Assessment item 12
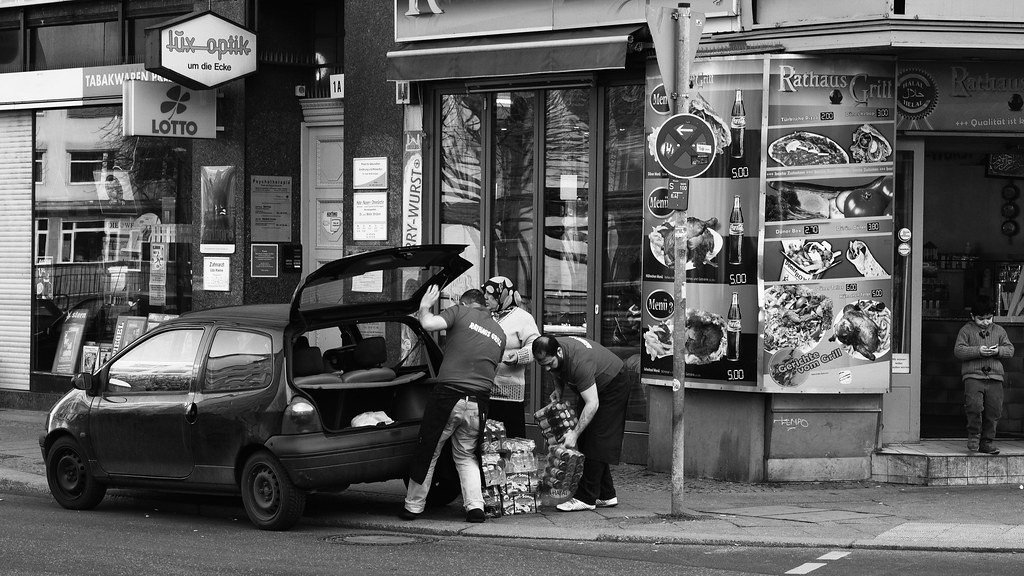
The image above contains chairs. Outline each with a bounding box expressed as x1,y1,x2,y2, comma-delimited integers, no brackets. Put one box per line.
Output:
290,335,387,376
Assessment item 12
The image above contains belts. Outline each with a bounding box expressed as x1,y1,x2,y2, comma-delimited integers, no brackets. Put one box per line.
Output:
461,395,479,403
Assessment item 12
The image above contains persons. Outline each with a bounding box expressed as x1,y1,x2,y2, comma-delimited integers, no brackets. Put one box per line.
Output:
532,334,631,511
953,294,1016,454
447,275,542,506
398,284,507,523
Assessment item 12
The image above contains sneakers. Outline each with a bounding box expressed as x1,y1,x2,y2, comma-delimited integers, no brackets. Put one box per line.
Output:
596,497,619,507
967,433,980,451
980,442,1000,454
556,498,596,511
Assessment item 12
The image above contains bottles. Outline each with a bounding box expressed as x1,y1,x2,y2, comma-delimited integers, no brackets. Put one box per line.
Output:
729,195,744,264
480,419,537,519
534,401,579,446
731,89,746,158
726,292,742,361
544,446,584,493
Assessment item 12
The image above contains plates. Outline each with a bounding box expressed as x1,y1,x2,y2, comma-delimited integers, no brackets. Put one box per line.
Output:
834,300,890,360
650,224,723,270
763,285,826,355
768,132,849,169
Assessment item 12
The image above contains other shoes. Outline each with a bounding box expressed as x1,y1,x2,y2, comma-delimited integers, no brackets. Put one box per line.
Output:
396,504,417,520
465,509,486,523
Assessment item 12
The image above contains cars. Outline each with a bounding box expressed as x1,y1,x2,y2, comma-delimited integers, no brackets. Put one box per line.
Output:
39,242,473,530
32,289,192,373
